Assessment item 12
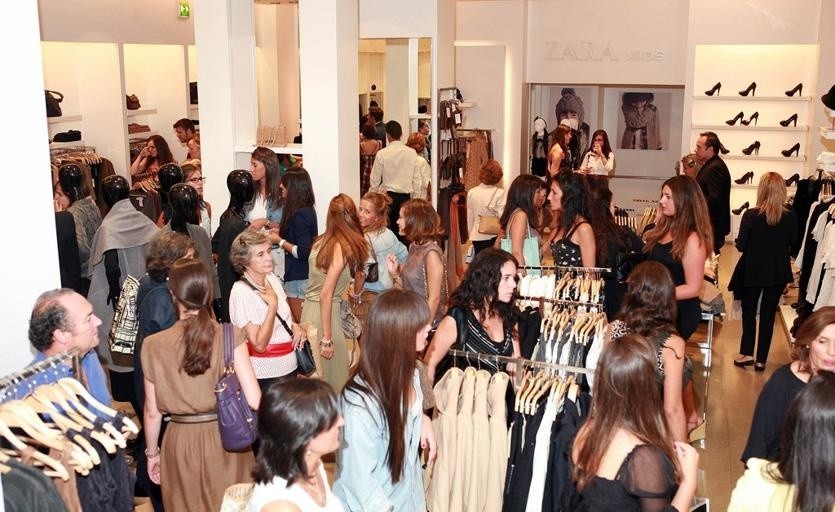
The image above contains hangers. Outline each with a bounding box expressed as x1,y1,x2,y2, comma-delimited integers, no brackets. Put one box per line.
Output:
614,206,658,233
50,144,102,167
442,267,608,424
130,170,158,195
0,350,140,483
817,171,835,225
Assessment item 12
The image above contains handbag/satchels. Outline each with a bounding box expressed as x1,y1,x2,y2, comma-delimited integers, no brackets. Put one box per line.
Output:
477,214,502,236
123,90,143,109
219,481,256,512
615,251,651,284
686,493,711,511
335,305,362,340
349,237,378,284
213,319,266,451
290,334,317,375
500,211,544,279
46,84,72,120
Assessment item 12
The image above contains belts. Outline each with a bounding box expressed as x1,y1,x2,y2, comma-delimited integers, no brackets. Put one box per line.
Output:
162,407,216,431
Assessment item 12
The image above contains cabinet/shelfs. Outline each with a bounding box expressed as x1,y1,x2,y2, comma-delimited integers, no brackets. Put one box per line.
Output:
127,109,160,140
692,96,812,218
47,113,86,149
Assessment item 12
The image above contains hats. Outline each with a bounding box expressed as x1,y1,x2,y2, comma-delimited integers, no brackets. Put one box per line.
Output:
556,88,585,131
821,84,834,111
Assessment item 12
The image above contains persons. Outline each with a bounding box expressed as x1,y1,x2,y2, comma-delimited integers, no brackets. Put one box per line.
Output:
3,101,835,512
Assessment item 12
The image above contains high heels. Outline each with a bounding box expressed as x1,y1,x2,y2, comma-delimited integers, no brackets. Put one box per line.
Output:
726,81,762,217
779,79,803,213
705,80,724,100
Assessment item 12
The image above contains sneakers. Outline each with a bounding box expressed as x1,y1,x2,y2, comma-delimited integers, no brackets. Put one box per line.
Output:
734,353,754,367
755,360,770,371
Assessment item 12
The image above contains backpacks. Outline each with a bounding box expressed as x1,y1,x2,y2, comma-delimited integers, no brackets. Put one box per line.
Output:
103,267,172,374
